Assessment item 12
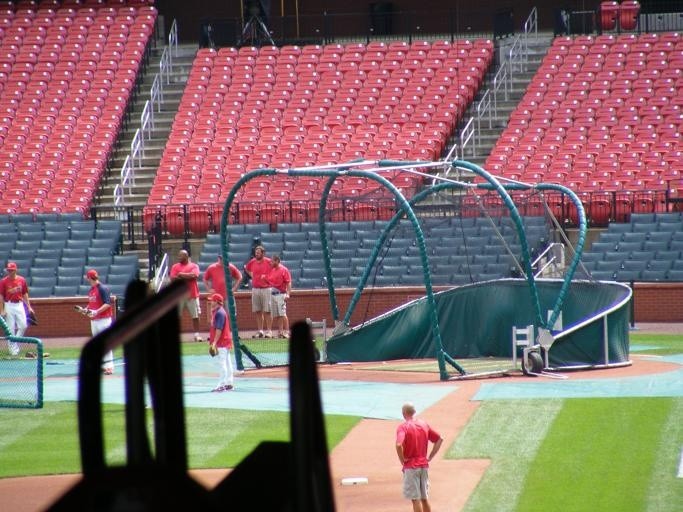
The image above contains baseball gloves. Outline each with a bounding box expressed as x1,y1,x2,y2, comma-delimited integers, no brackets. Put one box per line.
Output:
25,311,38,326
208,342,218,357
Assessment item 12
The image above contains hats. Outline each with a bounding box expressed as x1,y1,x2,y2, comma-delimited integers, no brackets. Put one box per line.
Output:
206,293,223,303
82,269,97,280
5,263,17,272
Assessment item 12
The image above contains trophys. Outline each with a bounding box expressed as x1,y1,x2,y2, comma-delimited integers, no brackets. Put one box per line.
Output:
71,304,89,317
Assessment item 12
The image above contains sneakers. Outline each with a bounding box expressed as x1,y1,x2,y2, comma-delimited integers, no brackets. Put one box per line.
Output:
193,330,291,343
211,385,234,393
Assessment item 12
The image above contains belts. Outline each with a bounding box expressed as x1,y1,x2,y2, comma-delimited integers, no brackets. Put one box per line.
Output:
271,291,285,295
3,299,20,303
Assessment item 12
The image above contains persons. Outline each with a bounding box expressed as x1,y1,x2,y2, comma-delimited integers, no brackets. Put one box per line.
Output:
262,254,292,339
168,249,204,342
79,269,114,375
0,261,36,357
241,245,276,339
205,292,235,393
394,402,444,512
201,251,242,341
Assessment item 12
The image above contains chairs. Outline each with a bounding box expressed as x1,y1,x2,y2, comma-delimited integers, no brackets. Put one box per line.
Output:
1,218,141,298
199,215,555,289
1,4,158,216
459,28,682,227
568,212,682,287
141,39,501,238
